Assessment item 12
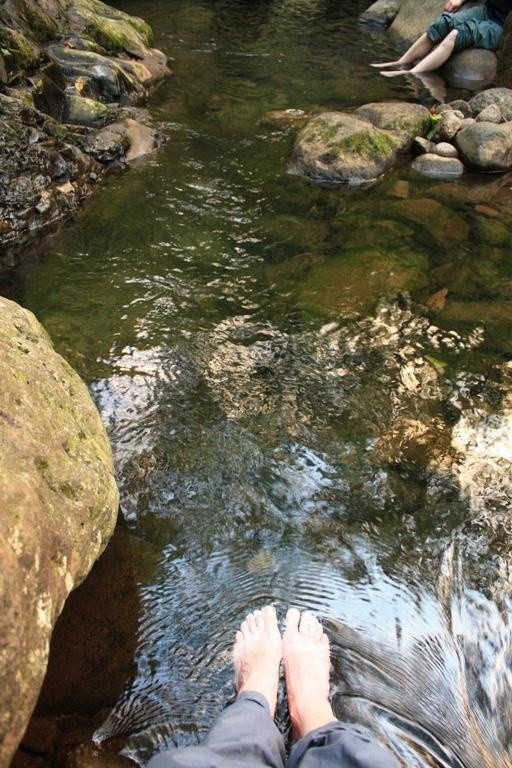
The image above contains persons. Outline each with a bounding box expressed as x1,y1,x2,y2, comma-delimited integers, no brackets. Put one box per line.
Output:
147,605,402,768
369,0,512,78
